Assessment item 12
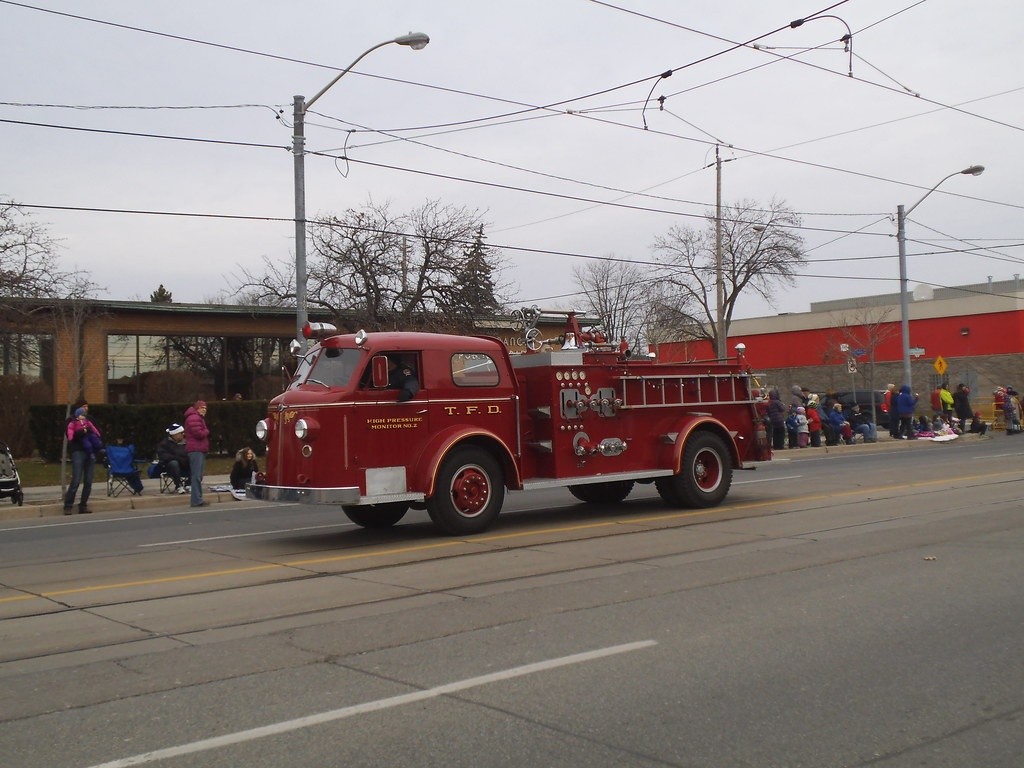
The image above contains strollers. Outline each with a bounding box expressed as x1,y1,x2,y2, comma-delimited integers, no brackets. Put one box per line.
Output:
0,442,24,507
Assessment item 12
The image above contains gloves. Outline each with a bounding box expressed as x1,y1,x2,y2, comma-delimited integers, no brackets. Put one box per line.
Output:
398,390,412,401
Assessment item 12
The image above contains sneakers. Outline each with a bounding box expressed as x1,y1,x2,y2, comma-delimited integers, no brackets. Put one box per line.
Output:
176,486,191,494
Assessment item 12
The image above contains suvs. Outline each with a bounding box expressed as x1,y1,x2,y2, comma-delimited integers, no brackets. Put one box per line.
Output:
819,389,893,429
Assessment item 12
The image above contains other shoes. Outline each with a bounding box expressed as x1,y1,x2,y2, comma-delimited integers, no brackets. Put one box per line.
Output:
864,438,876,443
907,437,918,440
90,454,95,459
191,501,209,507
980,435,988,439
64,506,72,514
1007,429,1024,434
846,439,855,445
100,449,105,453
79,506,92,513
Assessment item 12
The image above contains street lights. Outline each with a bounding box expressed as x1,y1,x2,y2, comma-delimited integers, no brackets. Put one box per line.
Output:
896,165,985,398
714,224,765,361
289,30,429,366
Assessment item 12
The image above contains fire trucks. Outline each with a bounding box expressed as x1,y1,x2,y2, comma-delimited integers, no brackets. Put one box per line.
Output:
244,305,776,536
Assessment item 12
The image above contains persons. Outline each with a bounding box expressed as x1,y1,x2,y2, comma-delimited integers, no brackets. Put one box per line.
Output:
159,422,192,494
880,383,988,439
184,400,211,507
62,400,106,515
767,385,877,450
992,384,1024,435
233,394,242,401
372,354,419,403
230,446,258,490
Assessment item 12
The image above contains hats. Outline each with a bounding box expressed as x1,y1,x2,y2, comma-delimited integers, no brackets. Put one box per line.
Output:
74,408,86,418
75,399,88,409
797,407,805,413
975,411,981,417
752,390,759,397
194,401,206,409
833,404,842,411
790,407,797,414
802,387,810,392
165,423,184,435
850,401,859,410
808,394,818,401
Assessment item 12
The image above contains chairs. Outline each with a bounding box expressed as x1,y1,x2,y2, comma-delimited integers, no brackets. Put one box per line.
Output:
160,453,200,494
103,457,143,496
